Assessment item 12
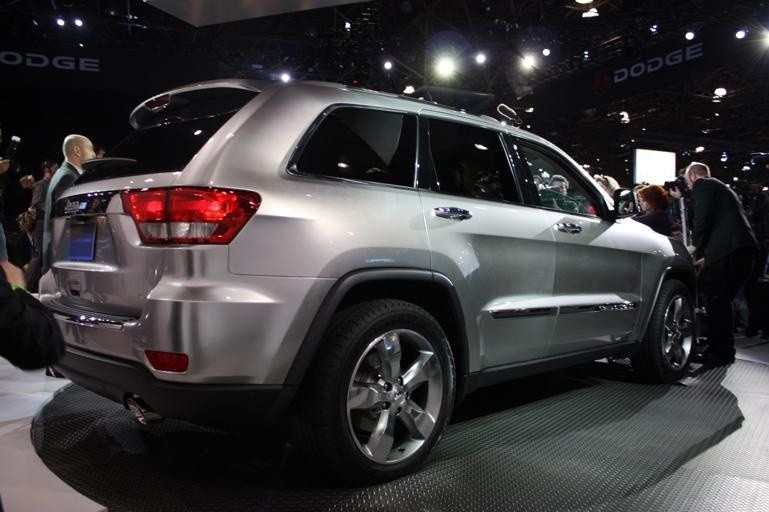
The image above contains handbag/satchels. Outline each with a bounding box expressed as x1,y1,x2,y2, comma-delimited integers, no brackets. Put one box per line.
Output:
2,268,66,371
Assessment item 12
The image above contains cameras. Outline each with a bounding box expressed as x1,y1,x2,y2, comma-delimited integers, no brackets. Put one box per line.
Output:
664,176,685,191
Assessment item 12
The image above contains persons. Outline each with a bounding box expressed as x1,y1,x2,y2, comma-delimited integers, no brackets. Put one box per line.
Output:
528,160,768,369
0,130,106,378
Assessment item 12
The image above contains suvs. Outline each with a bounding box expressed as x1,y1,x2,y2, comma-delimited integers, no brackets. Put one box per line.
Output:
40,76,700,481
